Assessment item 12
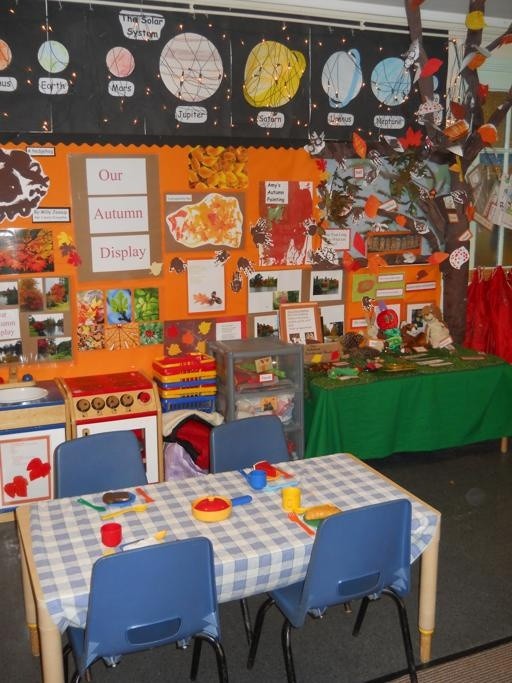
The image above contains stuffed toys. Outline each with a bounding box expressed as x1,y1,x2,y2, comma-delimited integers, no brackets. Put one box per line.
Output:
421,302,455,351
376,300,405,355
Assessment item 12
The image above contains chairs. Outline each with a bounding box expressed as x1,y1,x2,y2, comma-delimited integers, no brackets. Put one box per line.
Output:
211,413,351,645
66,538,228,682
248,497,418,682
55,430,186,682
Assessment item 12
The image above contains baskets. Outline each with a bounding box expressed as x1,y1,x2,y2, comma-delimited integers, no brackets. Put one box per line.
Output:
152,353,219,412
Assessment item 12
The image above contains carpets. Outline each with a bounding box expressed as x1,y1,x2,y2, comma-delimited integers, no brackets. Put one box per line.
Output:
371,631,512,682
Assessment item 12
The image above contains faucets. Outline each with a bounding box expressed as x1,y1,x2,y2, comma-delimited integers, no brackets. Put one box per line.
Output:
8,366,18,383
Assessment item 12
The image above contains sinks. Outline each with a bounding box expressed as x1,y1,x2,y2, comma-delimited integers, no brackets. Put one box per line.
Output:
0,386,49,404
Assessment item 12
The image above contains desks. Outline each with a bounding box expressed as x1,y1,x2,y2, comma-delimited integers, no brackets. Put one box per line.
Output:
16,452,442,683
305,343,512,463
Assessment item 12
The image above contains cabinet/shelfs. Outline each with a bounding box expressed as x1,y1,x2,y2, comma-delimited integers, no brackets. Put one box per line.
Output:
209,339,305,461
